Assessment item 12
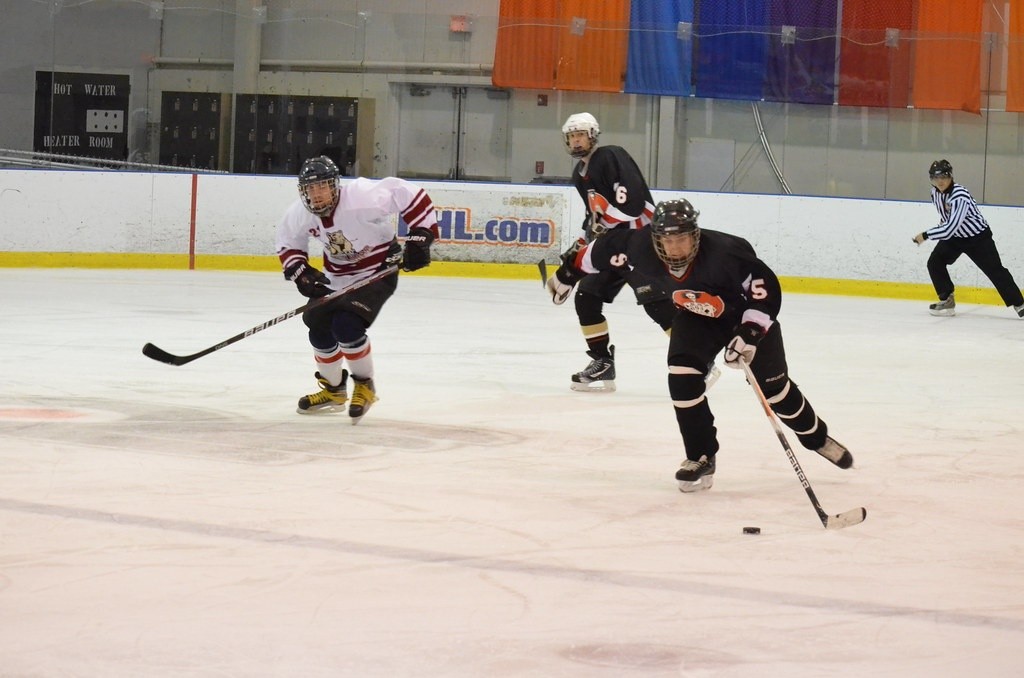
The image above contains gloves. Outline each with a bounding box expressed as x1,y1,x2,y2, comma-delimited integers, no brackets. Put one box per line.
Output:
724,321,767,370
402,227,433,272
284,261,337,298
547,262,579,304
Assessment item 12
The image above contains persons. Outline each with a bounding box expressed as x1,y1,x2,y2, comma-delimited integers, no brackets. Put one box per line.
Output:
546,198,853,482
275,155,440,419
915,159,1024,317
561,112,716,383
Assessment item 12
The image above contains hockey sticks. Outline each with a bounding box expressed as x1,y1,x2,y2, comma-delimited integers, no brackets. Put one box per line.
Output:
737,353,867,529
742,527,760,535
142,262,405,366
538,259,547,288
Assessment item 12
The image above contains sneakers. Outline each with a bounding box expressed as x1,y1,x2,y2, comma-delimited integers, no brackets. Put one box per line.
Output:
297,369,348,414
676,454,716,493
1014,302,1024,319
571,345,616,393
929,294,955,316
815,436,860,470
348,374,376,425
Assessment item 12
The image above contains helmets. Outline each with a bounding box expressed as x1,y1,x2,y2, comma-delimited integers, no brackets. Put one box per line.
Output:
929,159,953,179
298,155,340,218
651,199,699,270
562,112,600,159
